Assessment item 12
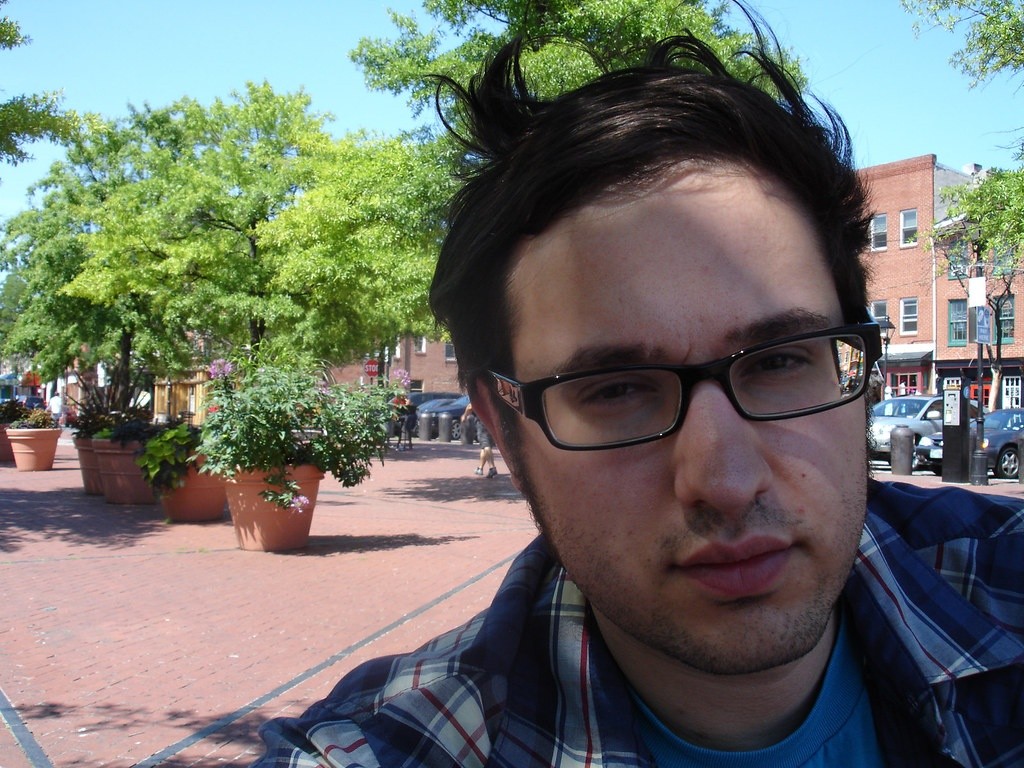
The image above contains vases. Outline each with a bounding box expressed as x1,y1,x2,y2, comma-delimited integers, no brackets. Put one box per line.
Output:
222,464,324,551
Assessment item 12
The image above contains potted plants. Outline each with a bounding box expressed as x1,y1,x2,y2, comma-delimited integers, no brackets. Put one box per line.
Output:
0,363,229,521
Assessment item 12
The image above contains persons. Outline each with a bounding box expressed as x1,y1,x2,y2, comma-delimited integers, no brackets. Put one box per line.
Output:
44,392,64,427
387,395,417,450
460,402,497,479
257,0,1024,768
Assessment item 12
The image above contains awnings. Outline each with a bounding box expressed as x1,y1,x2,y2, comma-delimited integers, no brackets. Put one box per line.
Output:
872,351,933,368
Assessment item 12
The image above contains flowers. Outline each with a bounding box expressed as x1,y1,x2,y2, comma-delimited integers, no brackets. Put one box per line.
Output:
186,336,411,513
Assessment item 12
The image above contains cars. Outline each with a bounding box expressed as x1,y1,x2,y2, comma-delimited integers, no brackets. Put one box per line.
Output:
383,392,470,440
916,407,1024,478
868,395,990,470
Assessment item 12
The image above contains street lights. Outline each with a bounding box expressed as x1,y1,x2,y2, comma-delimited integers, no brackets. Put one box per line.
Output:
878,316,895,405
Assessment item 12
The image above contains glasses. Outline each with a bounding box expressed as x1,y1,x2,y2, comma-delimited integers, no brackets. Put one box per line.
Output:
483,320,882,451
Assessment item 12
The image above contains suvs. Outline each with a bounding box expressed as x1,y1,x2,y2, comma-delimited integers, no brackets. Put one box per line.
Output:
17,395,47,413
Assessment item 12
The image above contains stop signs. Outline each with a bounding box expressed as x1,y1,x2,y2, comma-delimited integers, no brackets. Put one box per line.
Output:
363,359,381,377
